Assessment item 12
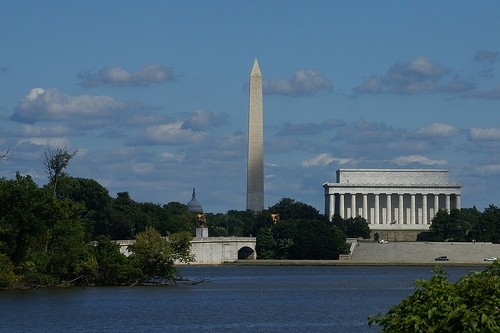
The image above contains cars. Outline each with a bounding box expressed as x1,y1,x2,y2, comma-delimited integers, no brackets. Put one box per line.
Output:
435,256,450,262
484,256,497,261
379,240,389,244
